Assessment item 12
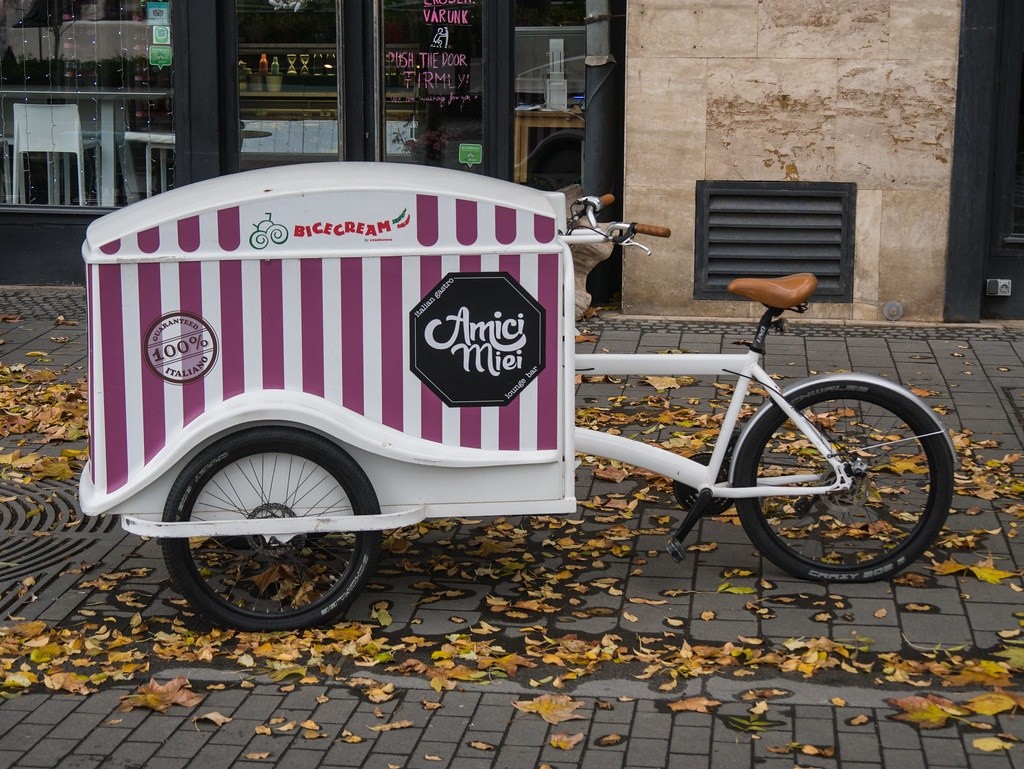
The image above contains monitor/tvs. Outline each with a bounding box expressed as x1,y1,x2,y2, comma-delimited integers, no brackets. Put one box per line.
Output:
513,26,588,112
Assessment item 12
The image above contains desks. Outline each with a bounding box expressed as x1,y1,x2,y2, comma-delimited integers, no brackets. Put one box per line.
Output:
514,109,586,184
0,86,172,208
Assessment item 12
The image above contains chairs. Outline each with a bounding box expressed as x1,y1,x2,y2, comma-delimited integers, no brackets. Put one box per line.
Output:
0,99,176,206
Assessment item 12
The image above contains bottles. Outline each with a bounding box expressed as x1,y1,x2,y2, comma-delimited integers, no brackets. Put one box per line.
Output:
271,57,279,74
258,53,268,74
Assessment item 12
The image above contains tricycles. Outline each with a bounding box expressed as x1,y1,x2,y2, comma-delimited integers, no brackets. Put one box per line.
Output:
79,160,958,630
